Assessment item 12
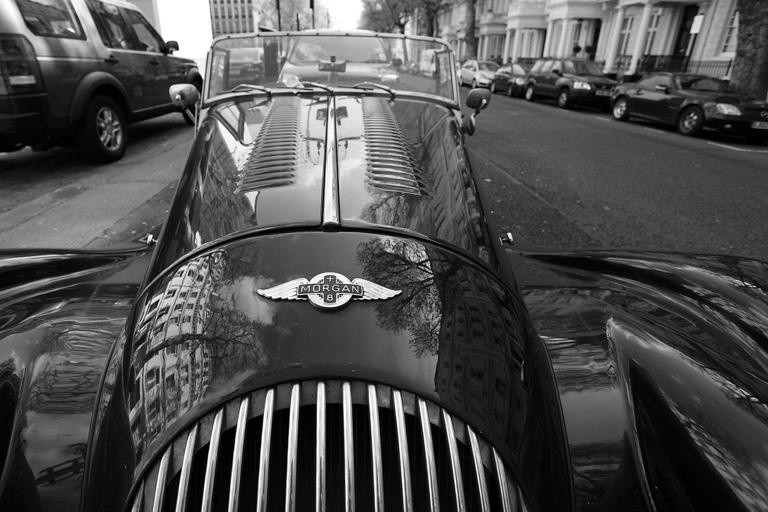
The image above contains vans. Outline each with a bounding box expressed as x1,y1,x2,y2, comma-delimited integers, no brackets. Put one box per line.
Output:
419,48,451,80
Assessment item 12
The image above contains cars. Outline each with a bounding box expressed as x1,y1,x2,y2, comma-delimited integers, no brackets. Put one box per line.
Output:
609,72,768,145
460,60,500,89
490,62,533,97
0,25,768,512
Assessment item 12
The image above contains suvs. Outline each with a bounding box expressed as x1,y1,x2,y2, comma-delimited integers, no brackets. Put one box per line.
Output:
0,1,205,165
525,57,617,113
